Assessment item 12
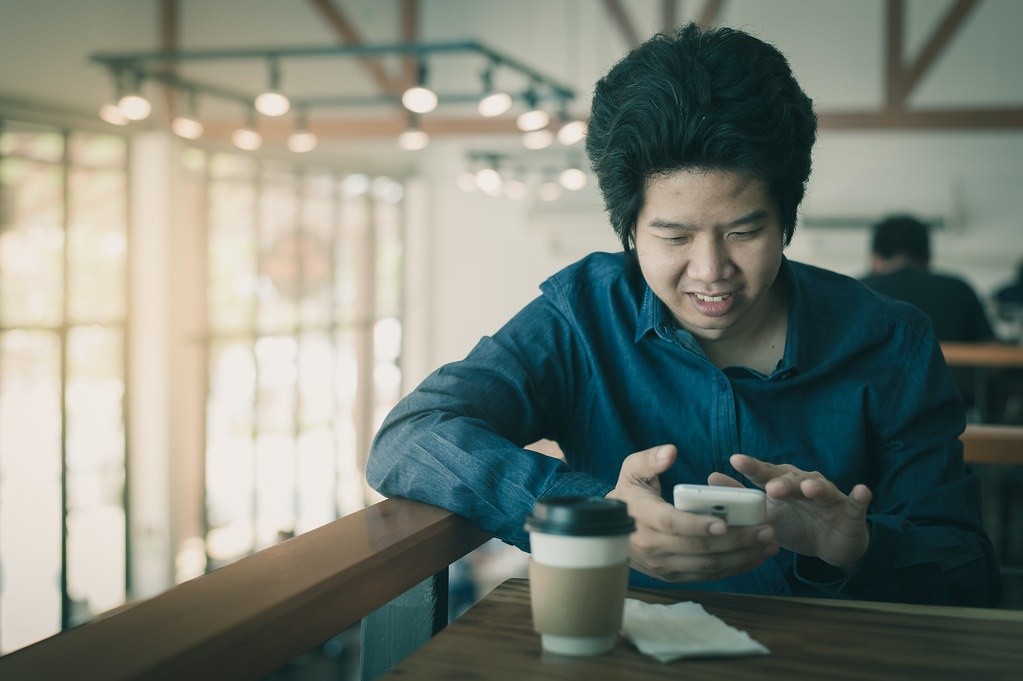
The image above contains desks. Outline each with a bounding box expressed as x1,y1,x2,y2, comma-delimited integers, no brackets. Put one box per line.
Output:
387,577,1023,681
938,339,1023,369
957,423,1023,464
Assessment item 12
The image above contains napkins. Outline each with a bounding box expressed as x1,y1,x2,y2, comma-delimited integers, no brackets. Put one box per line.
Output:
620,593,774,664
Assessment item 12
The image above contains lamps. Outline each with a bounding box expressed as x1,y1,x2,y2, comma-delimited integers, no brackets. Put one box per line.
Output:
515,76,550,131
398,59,440,118
253,57,292,118
551,90,590,148
473,60,514,118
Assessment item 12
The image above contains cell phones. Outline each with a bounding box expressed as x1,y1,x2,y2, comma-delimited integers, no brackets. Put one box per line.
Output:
673,484,767,528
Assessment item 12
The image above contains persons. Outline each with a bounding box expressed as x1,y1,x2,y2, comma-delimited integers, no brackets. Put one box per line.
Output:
861,214,992,422
367,22,998,607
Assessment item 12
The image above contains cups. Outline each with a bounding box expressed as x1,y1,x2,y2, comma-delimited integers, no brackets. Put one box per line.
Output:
524,495,636,655
